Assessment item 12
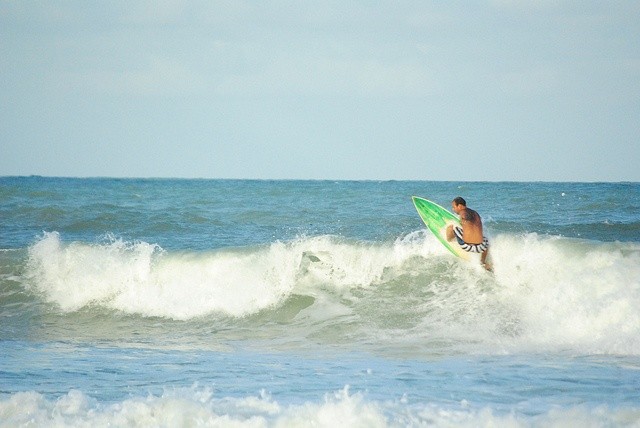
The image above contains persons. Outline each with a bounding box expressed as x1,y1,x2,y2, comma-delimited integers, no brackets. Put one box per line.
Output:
446,196,489,266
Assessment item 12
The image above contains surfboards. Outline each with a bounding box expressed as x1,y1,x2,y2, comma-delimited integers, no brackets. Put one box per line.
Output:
412,196,490,266
